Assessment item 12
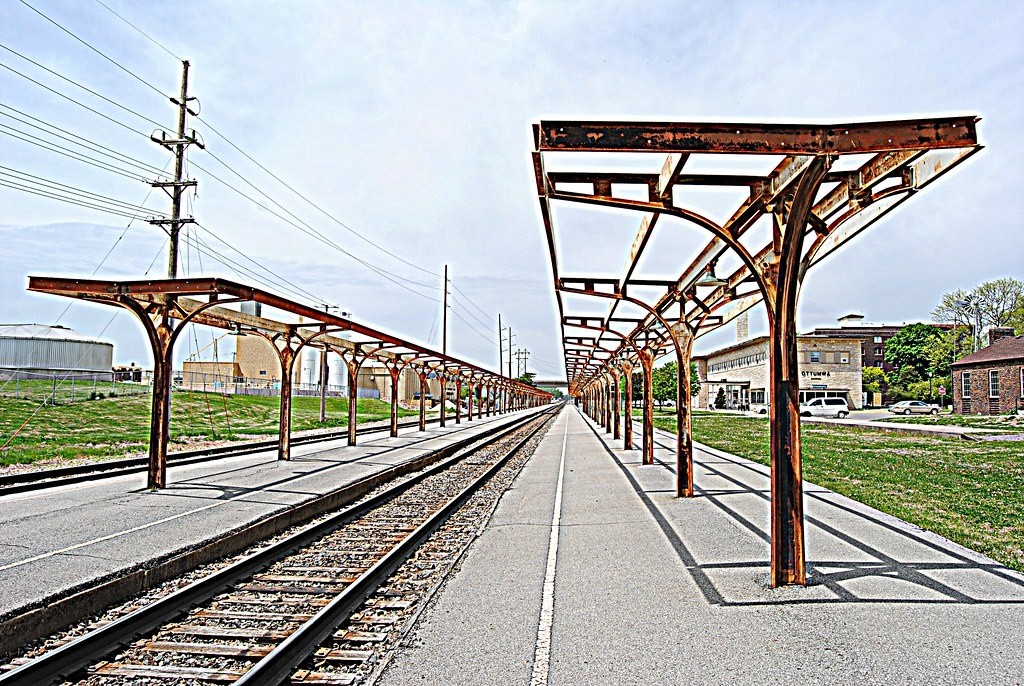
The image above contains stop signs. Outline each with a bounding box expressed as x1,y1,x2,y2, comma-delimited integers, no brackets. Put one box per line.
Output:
939,387,946,395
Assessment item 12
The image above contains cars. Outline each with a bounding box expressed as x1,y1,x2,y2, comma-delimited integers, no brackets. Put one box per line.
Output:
655,399,675,407
753,402,771,415
889,401,943,415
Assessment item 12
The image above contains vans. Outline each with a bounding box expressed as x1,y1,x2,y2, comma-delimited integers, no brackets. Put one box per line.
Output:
800,397,851,418
